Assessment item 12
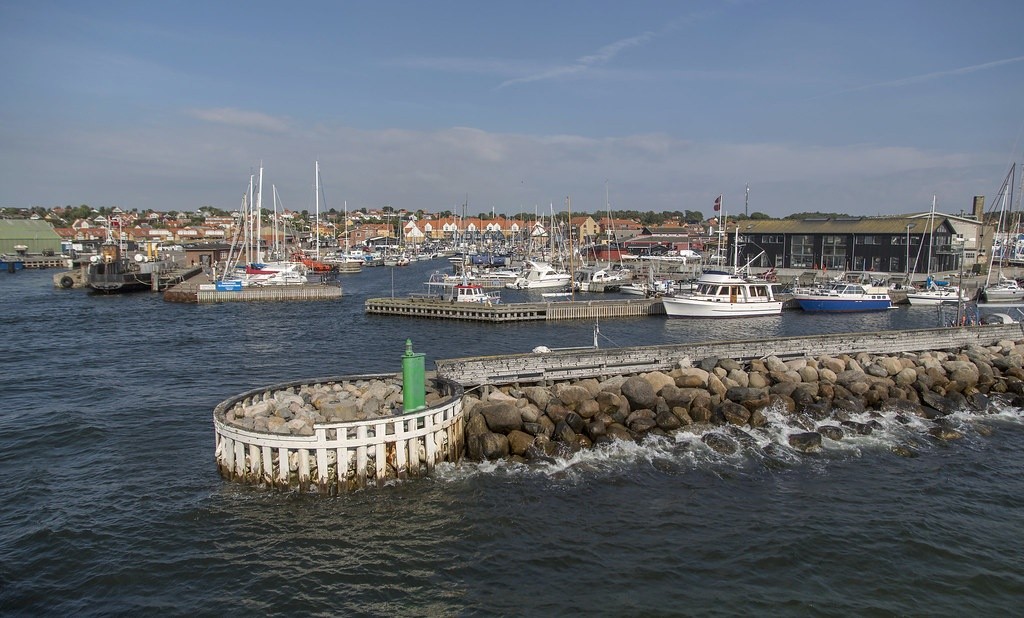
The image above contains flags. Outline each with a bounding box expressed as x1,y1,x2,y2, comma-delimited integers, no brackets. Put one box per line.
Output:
714,204,720,211
715,196,721,203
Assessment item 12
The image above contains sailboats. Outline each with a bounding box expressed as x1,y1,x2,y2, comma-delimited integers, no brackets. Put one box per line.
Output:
971,184,1024,303
904,195,970,305
206,157,933,319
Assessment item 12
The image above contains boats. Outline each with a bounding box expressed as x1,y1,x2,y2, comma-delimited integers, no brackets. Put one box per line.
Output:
84,214,179,294
